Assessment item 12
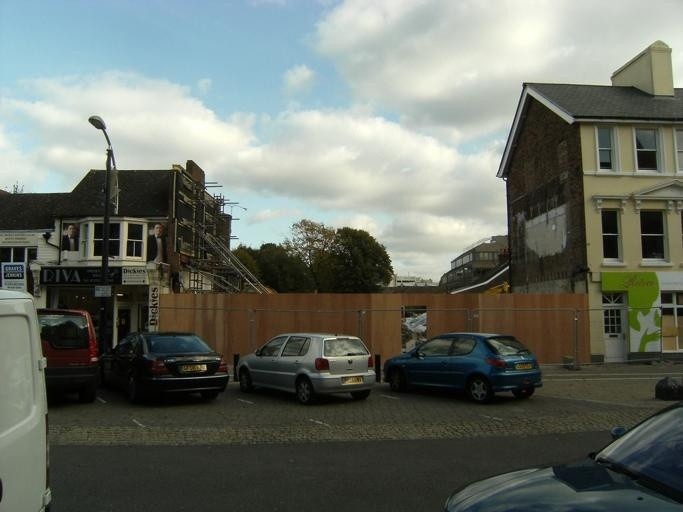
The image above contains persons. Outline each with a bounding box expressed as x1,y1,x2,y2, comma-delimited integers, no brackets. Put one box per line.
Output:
147,223,167,263
62,224,78,251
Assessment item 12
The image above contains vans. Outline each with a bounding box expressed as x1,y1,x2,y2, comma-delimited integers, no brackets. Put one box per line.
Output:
0,288,103,512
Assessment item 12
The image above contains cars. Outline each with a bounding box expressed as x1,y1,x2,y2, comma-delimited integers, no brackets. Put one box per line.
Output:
235,332,376,405
440,395,683,511
97,330,230,406
384,332,544,404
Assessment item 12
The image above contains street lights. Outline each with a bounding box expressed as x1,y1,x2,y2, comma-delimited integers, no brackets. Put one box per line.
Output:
89,118,115,395
